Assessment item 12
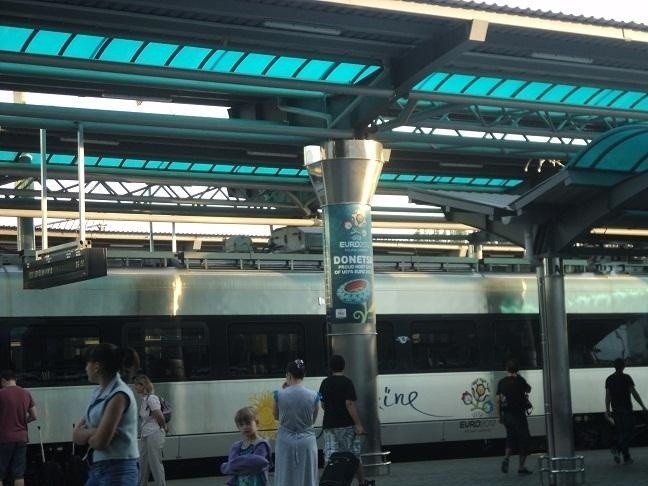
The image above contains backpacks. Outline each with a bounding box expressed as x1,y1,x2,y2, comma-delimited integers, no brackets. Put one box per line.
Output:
146,392,172,426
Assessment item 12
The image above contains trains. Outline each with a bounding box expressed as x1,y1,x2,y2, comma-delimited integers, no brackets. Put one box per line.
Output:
1,270,647,465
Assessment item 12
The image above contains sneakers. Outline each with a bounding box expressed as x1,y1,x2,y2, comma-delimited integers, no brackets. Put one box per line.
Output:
611,446,620,464
518,468,532,474
502,457,509,473
358,479,375,486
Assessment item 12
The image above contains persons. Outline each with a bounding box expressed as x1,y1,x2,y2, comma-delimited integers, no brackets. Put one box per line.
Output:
69,341,144,485
132,374,171,486
0,369,39,486
269,357,321,485
317,354,376,486
603,357,648,465
494,358,535,476
220,405,272,486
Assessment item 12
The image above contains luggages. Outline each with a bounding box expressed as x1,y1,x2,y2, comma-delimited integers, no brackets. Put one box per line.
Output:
319,451,360,486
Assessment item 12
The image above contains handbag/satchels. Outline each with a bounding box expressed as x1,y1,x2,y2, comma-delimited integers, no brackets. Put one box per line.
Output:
524,395,533,416
